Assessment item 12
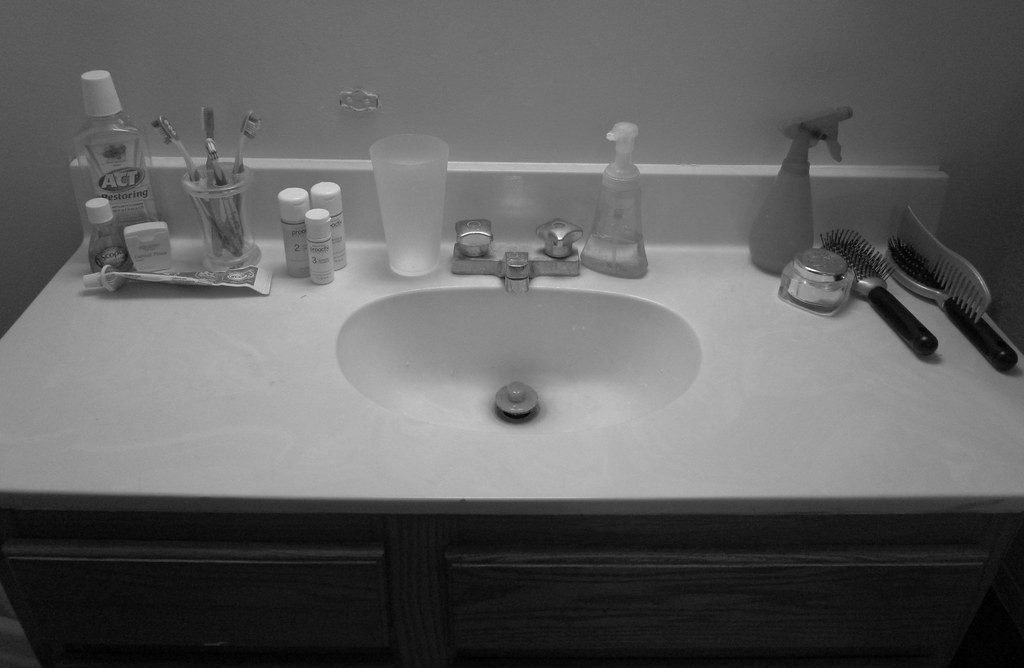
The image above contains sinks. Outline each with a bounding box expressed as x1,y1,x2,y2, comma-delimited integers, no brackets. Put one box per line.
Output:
333,286,705,436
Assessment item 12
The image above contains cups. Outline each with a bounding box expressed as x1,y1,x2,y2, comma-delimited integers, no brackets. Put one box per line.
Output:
181,161,262,274
368,132,449,277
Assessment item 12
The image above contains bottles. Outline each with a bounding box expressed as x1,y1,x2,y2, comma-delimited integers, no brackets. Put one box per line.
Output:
85,197,134,273
310,181,347,271
277,187,312,278
305,208,335,285
72,68,159,256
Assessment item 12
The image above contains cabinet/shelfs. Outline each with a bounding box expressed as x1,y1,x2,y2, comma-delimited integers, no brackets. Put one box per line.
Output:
0,512,1024,668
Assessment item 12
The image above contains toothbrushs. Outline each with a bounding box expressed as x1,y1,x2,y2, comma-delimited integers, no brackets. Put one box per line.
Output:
203,137,245,250
199,105,224,257
230,110,262,213
150,114,244,258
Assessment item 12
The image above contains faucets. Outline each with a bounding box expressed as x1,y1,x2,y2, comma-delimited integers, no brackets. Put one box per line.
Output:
450,218,584,296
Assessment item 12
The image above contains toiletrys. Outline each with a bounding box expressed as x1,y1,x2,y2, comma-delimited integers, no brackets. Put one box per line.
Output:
123,220,175,274
580,121,649,279
74,70,163,240
82,264,274,295
85,197,134,273
